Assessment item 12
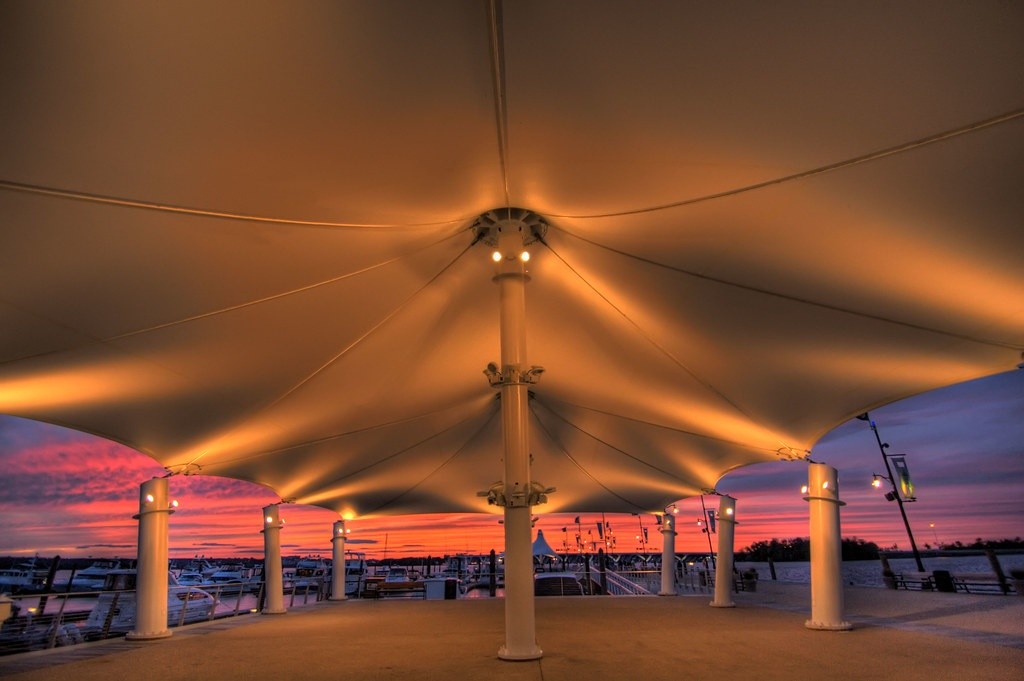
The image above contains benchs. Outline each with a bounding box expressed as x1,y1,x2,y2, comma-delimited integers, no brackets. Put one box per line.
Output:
893,570,937,592
952,572,1008,595
375,582,426,600
698,569,757,591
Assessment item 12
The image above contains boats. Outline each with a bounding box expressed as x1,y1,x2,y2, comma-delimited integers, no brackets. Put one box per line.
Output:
40,550,504,649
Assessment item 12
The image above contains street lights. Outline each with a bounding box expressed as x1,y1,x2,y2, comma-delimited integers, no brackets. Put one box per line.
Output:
871,471,934,590
929,522,940,548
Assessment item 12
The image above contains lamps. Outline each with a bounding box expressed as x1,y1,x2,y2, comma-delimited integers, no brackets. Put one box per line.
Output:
487,361,502,376
527,365,545,375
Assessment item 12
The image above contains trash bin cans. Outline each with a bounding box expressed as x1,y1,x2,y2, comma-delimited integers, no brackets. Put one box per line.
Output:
444,579,458,600
883,576,898,589
932,570,955,593
742,568,759,592
698,570,710,587
